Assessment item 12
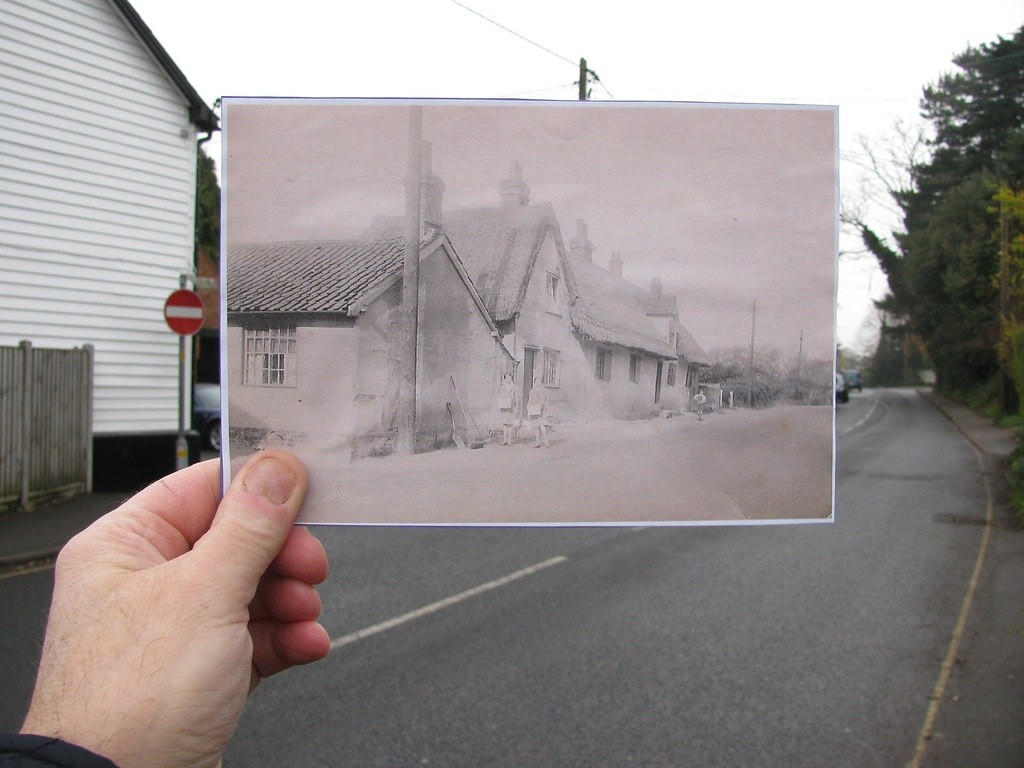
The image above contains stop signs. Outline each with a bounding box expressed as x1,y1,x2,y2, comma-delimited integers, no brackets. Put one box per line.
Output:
164,288,206,336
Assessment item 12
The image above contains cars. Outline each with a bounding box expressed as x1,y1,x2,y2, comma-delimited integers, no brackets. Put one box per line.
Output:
836,374,850,403
843,370,864,393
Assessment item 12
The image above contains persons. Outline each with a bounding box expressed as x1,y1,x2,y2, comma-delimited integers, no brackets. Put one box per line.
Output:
694,389,706,420
497,371,517,446
0,452,331,768
528,378,551,449
